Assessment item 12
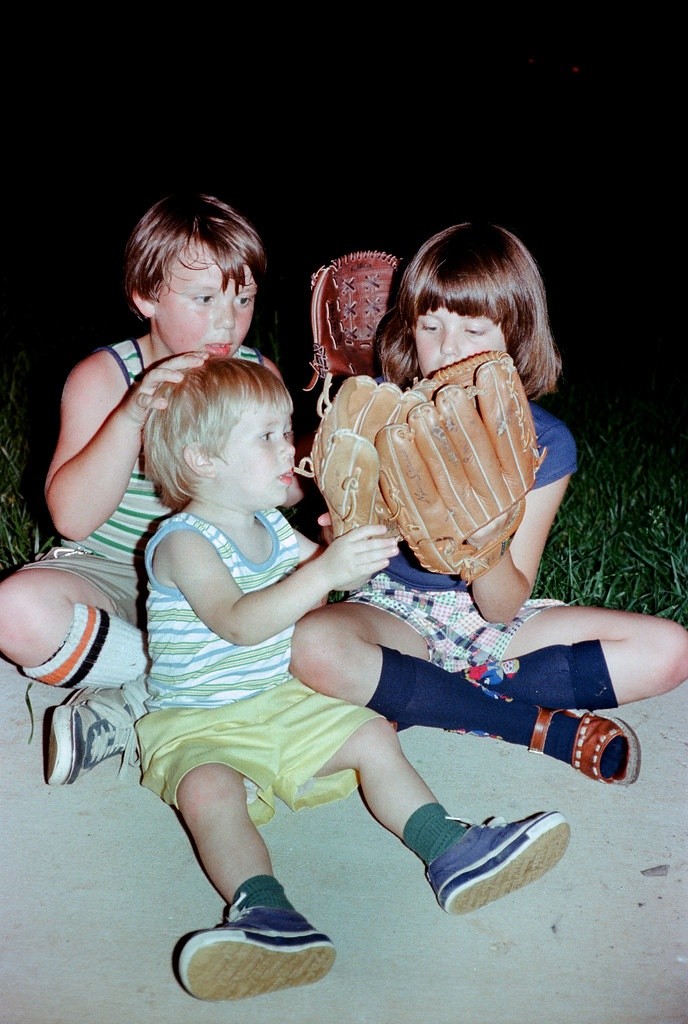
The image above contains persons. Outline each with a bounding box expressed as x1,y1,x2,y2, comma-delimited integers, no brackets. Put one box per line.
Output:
288,219,688,785
133,357,570,1001
0,197,304,785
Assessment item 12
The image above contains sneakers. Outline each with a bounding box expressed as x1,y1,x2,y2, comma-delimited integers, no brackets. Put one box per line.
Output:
45,674,149,786
424,808,571,916
178,892,335,1001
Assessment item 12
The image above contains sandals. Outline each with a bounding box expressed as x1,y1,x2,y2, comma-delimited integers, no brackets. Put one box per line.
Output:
526,704,642,787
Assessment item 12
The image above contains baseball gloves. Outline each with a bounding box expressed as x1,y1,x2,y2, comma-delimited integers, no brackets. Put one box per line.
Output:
309,348,546,587
